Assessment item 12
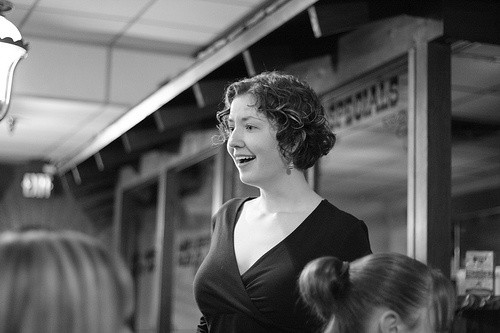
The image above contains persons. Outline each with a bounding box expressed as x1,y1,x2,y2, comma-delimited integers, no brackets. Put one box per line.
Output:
298,250,457,333
193,72,374,333
